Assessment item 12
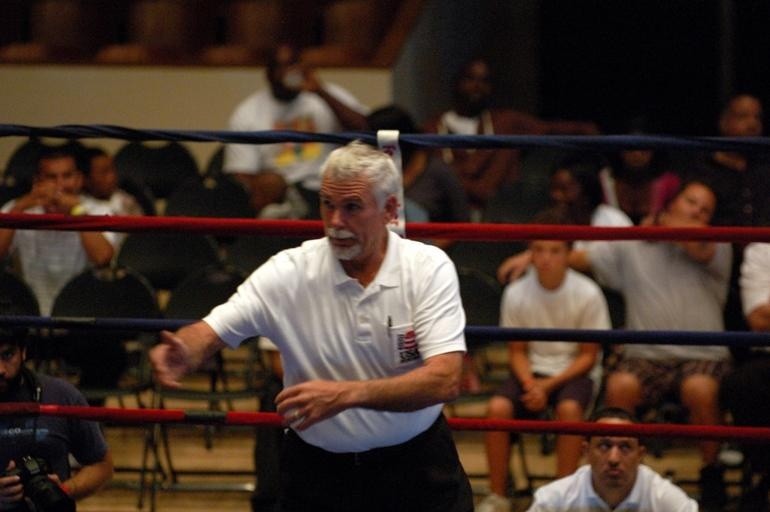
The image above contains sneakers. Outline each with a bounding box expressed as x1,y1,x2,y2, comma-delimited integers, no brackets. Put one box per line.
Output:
475,493,512,512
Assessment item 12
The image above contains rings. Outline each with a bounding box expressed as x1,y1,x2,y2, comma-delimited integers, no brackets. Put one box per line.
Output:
292,409,302,421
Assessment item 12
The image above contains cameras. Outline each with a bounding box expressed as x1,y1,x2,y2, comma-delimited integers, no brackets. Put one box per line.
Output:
1,456,76,512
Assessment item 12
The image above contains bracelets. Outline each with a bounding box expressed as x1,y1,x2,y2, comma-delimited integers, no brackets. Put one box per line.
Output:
68,204,86,217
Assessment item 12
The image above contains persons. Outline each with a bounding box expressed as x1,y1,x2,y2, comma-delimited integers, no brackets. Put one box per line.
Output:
220,29,368,381
0,324,115,512
693,90,768,361
736,240,770,478
494,174,734,478
360,105,476,255
417,49,674,221
474,210,614,512
148,144,475,512
78,149,158,349
0,148,140,390
529,407,701,512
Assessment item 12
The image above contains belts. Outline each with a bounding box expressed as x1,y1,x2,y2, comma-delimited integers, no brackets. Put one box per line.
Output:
283,410,444,463
